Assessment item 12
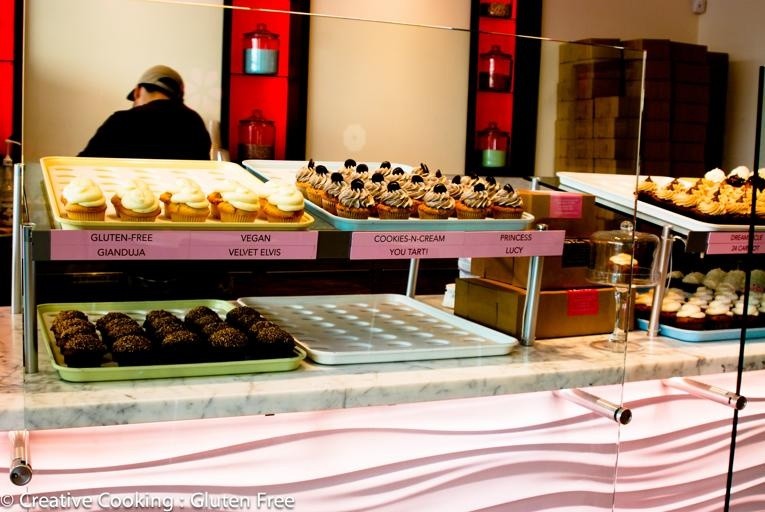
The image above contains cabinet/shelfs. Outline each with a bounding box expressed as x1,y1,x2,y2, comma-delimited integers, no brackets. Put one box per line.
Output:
0,157,765,430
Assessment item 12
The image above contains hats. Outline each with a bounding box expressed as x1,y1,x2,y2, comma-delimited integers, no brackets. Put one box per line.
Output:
127,64,185,102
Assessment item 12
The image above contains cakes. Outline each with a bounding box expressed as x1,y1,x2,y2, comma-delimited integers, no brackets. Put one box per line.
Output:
376,181,415,219
635,265,764,326
63,334,101,367
160,173,209,222
112,334,152,366
208,327,248,360
418,183,455,220
254,327,295,360
62,180,107,222
161,328,198,364
338,159,496,187
490,183,523,220
294,158,316,198
260,174,304,222
608,252,638,276
305,166,333,206
335,179,375,219
50,304,280,330
208,178,260,222
455,183,489,220
638,172,765,218
111,177,161,223
319,173,347,212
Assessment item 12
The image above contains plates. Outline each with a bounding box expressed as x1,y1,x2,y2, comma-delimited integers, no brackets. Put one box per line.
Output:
235,292,519,367
634,316,765,344
35,298,306,382
37,156,310,232
242,158,534,230
554,169,764,234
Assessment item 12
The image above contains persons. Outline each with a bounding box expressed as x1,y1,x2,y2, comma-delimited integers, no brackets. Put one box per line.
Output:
76,64,212,161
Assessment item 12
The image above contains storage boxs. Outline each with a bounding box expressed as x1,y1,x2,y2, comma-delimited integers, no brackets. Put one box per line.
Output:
555,36,728,173
